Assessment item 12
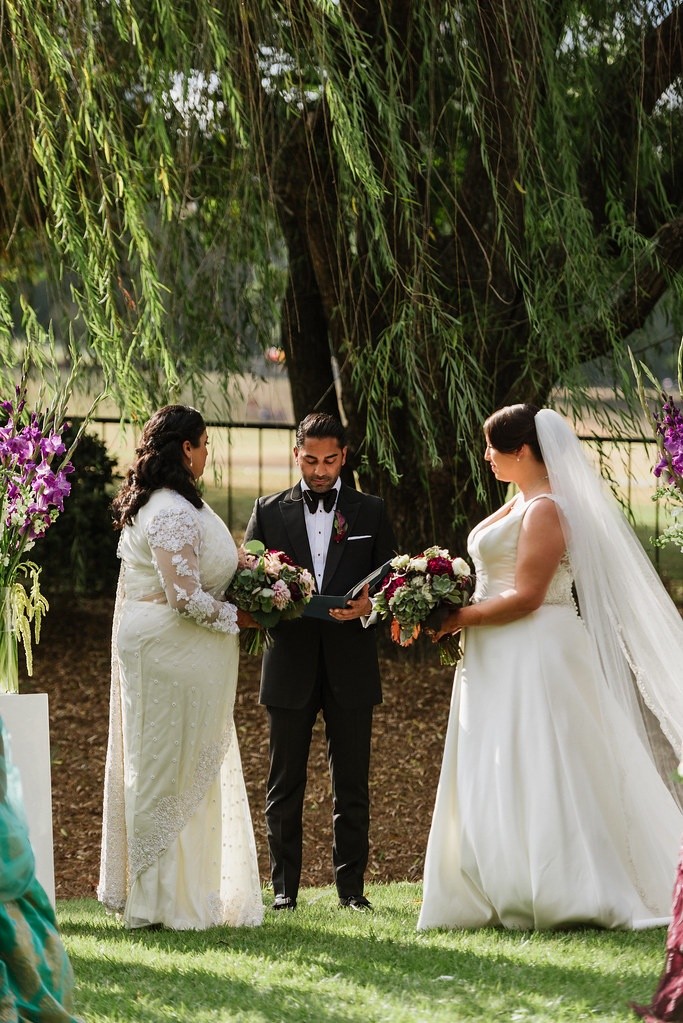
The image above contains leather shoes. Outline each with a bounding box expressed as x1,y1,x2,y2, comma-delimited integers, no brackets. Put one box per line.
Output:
339,896,374,914
271,895,297,911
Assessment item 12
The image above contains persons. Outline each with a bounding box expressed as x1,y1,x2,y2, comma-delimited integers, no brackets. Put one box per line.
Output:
240,412,401,913
99,403,265,885
413,404,683,935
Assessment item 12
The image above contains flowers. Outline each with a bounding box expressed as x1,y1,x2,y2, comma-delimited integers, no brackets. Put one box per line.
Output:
379,543,471,668
620,342,683,558
231,544,319,655
0,350,109,694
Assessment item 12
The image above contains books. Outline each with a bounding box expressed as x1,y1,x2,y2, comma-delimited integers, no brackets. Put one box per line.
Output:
302,556,395,625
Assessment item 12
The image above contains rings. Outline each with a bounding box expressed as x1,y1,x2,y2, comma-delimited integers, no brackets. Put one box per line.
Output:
429,629,433,636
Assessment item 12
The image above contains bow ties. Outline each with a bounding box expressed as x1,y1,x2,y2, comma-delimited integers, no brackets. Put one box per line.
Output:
303,489,338,515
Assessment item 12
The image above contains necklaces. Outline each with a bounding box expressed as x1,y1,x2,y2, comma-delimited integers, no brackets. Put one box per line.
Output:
510,473,548,512
